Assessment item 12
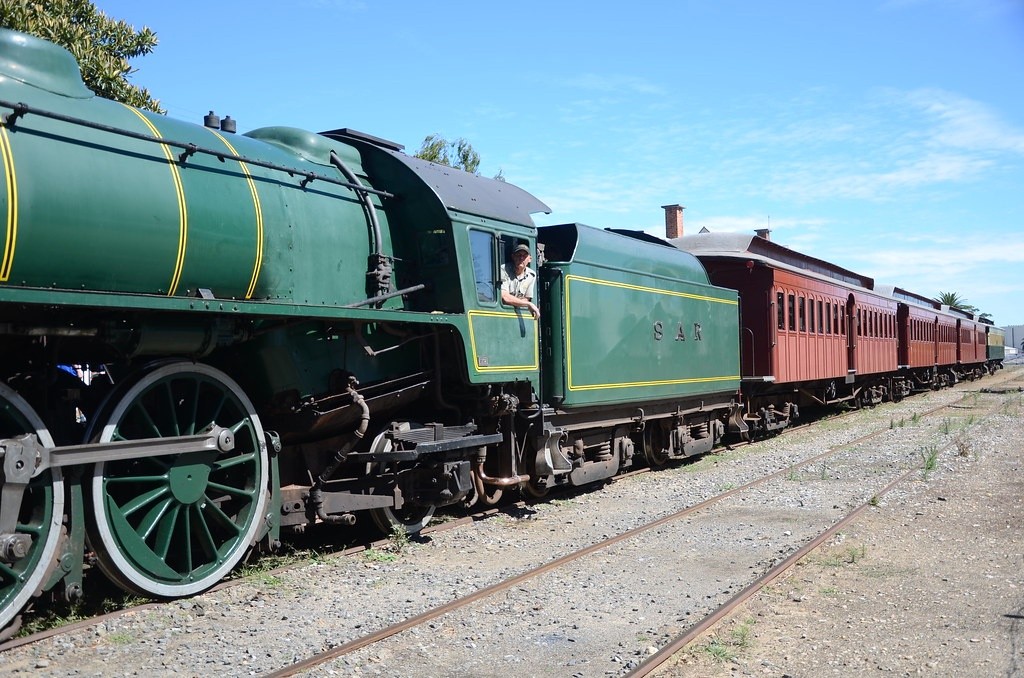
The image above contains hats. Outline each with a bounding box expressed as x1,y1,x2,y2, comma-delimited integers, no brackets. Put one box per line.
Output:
513,244,529,254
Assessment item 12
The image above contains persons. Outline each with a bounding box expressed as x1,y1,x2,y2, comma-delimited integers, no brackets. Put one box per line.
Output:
501,244,540,320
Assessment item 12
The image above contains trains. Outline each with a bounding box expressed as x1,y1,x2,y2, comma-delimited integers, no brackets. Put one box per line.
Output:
0,27,1007,635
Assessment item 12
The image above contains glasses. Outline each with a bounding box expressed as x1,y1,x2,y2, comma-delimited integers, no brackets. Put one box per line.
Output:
515,252,528,258
